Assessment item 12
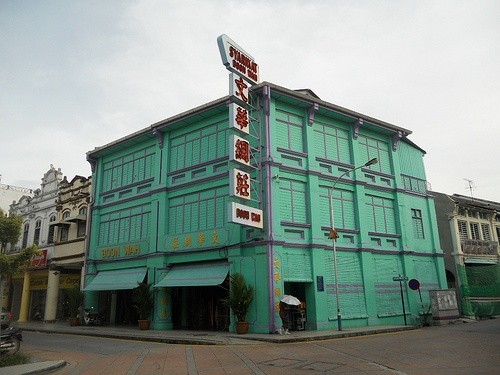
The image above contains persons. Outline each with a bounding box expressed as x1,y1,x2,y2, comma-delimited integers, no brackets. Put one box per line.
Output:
99,309,105,327
277,302,291,336
126,310,137,329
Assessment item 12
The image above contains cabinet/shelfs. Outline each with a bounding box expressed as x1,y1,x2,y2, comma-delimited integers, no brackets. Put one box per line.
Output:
291,310,307,330
208,293,230,331
100,293,112,322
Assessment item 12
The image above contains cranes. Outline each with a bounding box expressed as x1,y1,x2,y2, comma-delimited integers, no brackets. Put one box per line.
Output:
0,174,35,194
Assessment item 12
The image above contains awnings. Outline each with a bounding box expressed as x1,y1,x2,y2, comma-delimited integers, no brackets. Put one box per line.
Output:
81,266,148,292
152,261,230,287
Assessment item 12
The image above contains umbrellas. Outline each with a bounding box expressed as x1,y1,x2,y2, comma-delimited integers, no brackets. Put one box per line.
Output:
279,294,302,307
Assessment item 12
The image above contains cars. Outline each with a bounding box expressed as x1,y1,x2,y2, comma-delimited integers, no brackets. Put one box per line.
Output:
1,306,12,328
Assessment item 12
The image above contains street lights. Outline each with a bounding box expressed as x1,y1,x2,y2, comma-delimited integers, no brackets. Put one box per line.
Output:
328,157,379,332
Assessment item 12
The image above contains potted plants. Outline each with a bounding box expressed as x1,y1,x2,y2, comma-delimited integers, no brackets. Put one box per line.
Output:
133,280,160,331
64,284,86,326
217,272,256,335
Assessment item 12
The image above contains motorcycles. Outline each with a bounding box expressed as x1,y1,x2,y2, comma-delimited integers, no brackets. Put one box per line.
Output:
0,326,24,356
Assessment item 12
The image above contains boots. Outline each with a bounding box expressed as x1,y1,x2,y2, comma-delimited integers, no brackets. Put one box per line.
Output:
284,329,291,334
278,327,283,336
